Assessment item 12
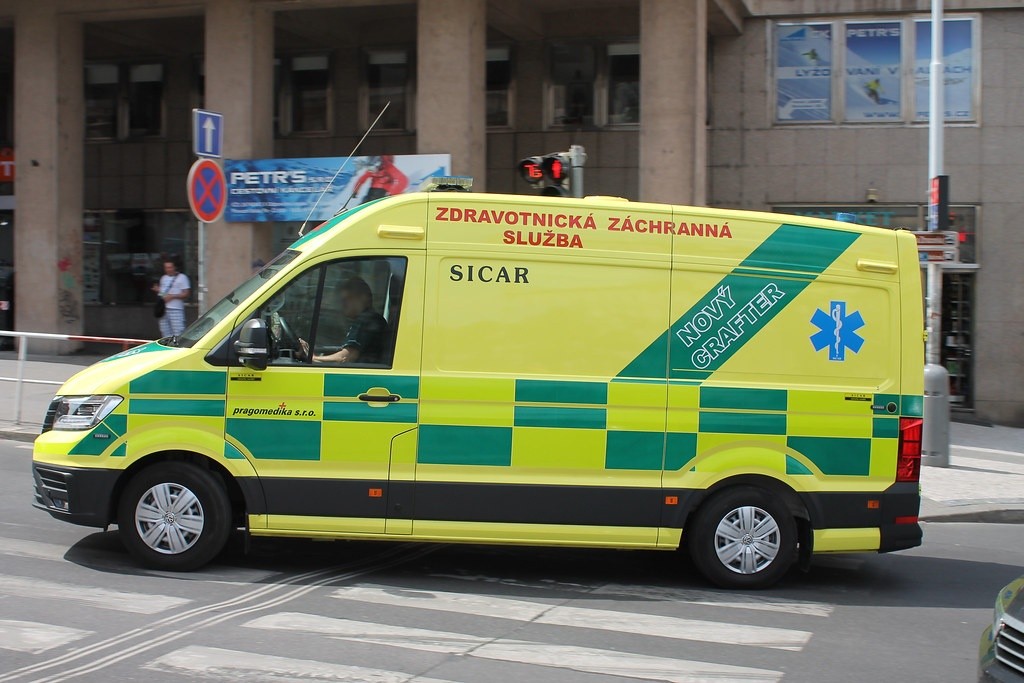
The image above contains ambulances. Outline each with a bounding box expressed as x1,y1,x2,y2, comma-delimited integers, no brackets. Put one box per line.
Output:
31,175,924,592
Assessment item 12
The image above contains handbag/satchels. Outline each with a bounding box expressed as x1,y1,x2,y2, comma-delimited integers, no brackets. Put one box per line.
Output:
154,295,166,317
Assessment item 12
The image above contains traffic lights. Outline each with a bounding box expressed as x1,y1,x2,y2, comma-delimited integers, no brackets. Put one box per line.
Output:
540,154,569,196
519,156,543,184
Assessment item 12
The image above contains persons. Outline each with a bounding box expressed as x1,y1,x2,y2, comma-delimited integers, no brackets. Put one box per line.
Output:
299,274,391,364
802,49,818,62
151,258,191,338
252,259,265,274
864,78,885,103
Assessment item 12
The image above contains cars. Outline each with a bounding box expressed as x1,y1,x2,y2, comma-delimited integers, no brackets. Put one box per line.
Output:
976,576,1024,683
